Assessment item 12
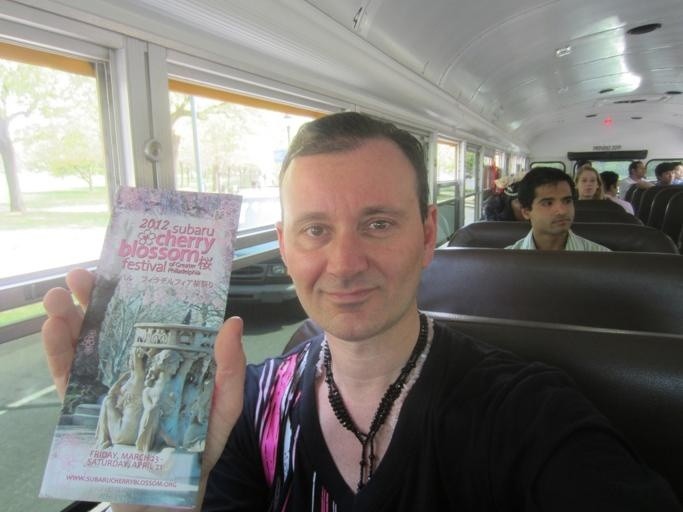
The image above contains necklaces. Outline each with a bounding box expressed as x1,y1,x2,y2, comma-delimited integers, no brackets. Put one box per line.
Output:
320,311,430,492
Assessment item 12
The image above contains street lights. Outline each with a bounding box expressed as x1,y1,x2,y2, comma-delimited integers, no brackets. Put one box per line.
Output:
282,113,292,144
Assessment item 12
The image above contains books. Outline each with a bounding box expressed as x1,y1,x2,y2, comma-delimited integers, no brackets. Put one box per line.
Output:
38,185,245,511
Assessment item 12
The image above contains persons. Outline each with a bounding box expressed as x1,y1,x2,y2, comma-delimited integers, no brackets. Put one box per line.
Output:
499,165,613,254
37,110,681,512
478,154,682,222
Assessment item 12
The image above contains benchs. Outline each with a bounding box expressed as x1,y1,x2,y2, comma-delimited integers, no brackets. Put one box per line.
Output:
416,181,683,511
